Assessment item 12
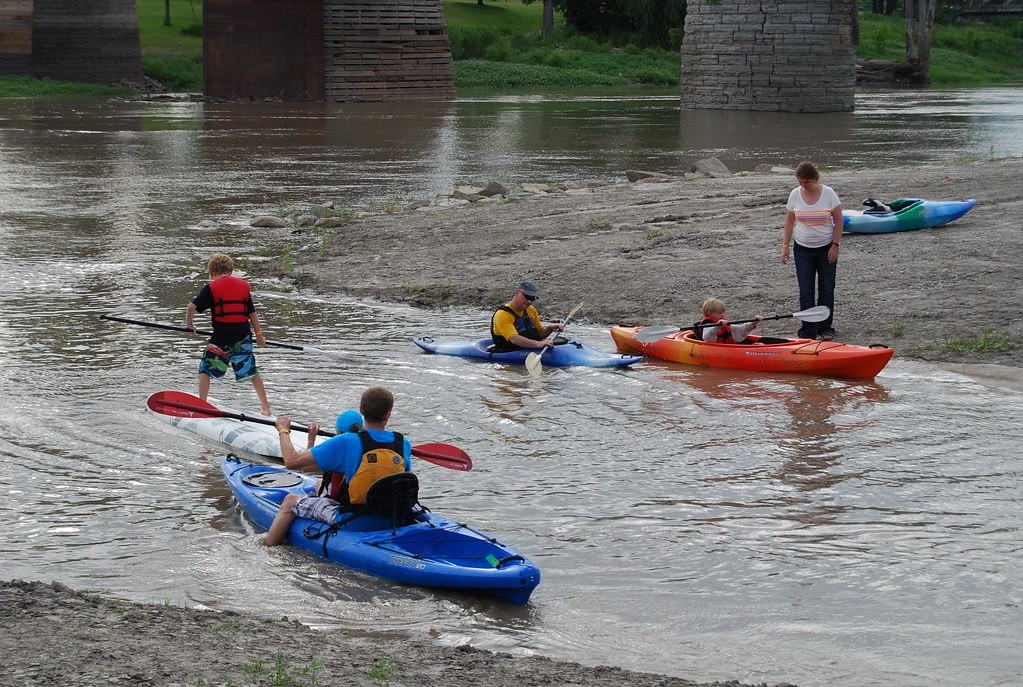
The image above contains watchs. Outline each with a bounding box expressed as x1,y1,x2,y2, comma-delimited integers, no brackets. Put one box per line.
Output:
832,243,839,247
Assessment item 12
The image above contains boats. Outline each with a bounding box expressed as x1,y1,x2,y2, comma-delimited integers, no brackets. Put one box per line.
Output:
832,197,977,233
608,322,895,380
219,451,541,604
412,335,644,369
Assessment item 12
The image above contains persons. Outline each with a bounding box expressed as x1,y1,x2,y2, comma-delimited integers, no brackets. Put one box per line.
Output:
780,160,843,341
490,282,564,353
700,297,765,345
186,252,271,416
261,386,412,547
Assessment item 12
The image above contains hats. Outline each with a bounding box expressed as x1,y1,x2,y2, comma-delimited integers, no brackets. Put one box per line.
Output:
518,282,540,299
337,409,363,434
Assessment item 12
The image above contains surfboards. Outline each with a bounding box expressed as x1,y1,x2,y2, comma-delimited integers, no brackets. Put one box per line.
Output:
146,392,330,459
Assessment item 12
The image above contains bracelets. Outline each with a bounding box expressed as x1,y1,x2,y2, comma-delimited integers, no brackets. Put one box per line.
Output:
548,326,554,332
256,331,262,336
783,245,788,248
279,429,289,435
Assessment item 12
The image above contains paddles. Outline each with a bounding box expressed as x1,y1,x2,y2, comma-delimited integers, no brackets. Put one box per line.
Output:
99,313,327,356
524,300,585,378
144,389,476,473
635,305,831,344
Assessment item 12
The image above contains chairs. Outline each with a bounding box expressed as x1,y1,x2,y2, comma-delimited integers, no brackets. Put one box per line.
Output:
335,472,418,533
691,322,702,340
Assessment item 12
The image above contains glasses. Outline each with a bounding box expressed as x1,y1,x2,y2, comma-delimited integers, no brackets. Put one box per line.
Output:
520,291,535,302
